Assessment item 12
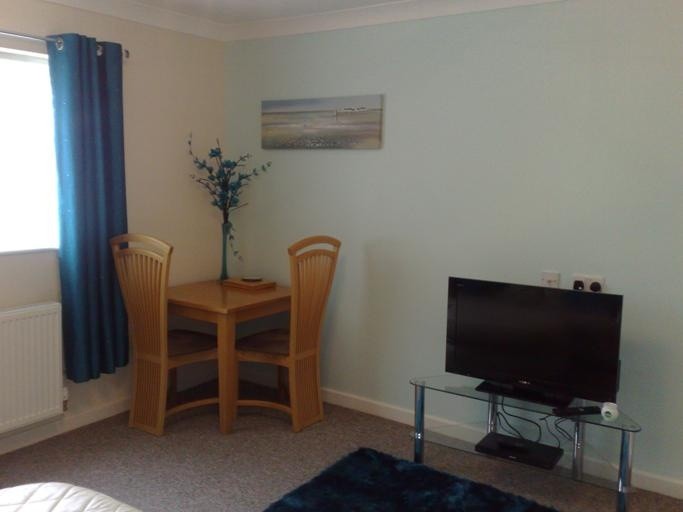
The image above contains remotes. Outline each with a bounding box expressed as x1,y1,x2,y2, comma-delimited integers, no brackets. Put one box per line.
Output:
552,405,600,416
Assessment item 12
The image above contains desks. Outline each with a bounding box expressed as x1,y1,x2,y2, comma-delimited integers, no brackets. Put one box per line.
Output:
410,373,640,512
168,279,290,435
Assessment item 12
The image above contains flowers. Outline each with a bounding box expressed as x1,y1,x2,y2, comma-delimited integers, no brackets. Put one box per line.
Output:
188,132,272,262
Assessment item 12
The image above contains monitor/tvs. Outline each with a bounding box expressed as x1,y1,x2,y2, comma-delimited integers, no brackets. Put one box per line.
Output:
445,276,623,408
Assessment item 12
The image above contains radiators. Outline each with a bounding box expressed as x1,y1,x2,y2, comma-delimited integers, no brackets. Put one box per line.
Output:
0,301,69,434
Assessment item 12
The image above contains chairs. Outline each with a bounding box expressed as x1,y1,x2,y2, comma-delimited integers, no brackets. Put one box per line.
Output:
110,233,218,437
236,235,341,433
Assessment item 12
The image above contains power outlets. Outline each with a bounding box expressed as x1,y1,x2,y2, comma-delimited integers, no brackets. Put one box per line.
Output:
590,277,607,293
572,274,590,292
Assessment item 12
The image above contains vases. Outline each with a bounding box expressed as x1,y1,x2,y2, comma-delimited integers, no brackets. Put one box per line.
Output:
220,222,230,284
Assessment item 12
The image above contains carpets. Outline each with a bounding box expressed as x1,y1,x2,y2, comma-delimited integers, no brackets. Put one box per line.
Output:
261,447,563,512
